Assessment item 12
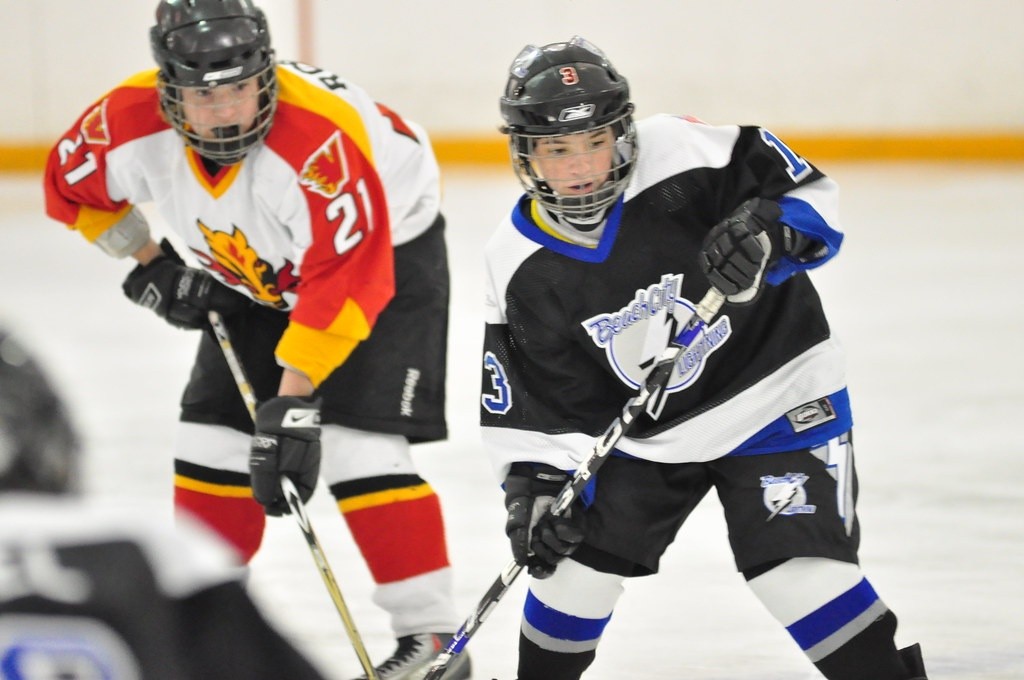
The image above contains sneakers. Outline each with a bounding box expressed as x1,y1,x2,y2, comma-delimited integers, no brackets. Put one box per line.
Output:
357,633,470,680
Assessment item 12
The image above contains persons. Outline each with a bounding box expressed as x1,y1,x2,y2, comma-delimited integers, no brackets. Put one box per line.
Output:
0,325,335,680
476,34,935,680
43,0,478,679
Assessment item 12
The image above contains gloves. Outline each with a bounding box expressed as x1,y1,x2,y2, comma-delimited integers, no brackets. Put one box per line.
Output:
506,486,583,579
699,196,781,306
249,394,324,517
121,237,243,328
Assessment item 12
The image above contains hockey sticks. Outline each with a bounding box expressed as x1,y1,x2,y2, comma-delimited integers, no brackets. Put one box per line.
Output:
209,305,380,680
424,231,776,680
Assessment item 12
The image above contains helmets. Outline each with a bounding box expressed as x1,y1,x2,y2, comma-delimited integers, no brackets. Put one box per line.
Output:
150,0,278,164
0,333,79,487
500,36,639,232
